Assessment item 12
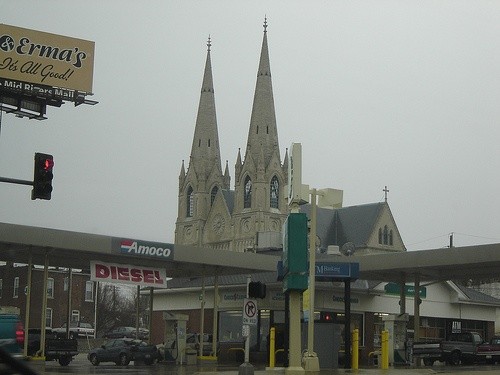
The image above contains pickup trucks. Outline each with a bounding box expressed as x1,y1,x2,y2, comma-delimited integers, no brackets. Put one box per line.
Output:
20,327,80,366
475,335,500,363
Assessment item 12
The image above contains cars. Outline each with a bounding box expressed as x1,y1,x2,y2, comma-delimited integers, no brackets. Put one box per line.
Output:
102,326,150,341
86,337,157,366
185,332,215,356
51,321,95,340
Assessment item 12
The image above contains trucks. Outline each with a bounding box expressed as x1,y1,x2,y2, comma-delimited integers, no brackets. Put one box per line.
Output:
416,332,488,363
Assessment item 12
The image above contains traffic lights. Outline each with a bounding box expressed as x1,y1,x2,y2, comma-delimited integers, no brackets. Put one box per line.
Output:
248,281,266,299
31,152,54,200
